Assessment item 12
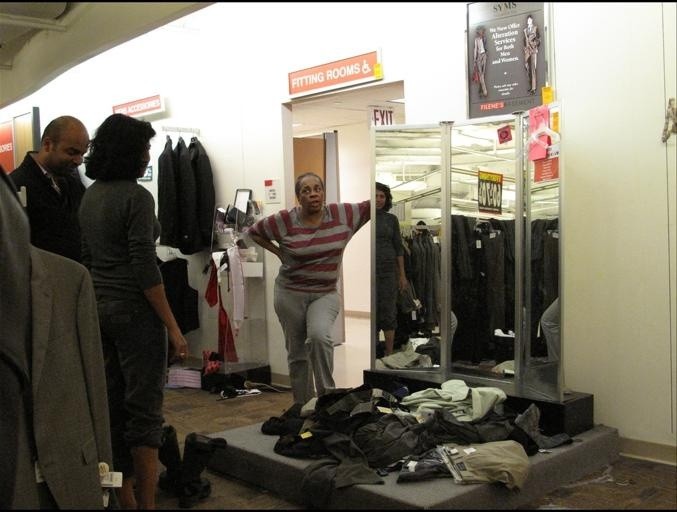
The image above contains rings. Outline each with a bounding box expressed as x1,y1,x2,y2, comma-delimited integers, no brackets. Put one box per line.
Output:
179,352,185,356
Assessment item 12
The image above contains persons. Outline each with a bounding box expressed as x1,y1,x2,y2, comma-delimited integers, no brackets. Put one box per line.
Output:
7,114,92,268
374,181,407,355
244,171,372,402
78,113,191,512
521,14,540,98
538,297,560,360
472,24,489,97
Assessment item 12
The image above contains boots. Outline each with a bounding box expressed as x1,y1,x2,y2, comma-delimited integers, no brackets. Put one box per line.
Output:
179,433,211,508
158,424,183,499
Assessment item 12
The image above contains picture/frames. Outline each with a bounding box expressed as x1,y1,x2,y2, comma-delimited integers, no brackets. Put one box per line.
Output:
465,2,559,124
11,106,41,169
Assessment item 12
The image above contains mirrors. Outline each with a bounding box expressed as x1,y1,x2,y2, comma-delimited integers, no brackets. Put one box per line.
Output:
447,111,525,398
520,106,563,403
370,121,447,384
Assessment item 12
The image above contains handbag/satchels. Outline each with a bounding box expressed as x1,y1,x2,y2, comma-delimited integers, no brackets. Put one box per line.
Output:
472,63,480,83
